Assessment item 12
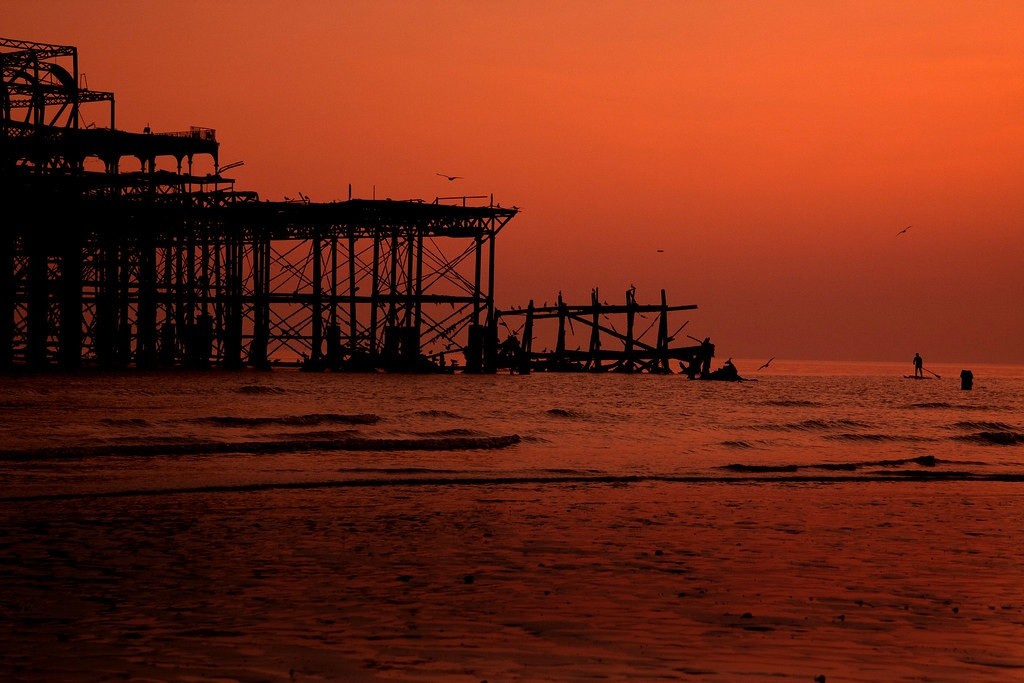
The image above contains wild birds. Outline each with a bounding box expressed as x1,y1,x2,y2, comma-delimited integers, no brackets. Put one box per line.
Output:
895,224,913,238
436,172,464,181
724,357,733,364
757,356,776,371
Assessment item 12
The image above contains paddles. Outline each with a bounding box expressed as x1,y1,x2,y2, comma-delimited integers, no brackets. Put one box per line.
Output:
922,367,942,379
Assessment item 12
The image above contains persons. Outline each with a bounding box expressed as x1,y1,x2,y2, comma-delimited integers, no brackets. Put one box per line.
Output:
913,353,923,377
